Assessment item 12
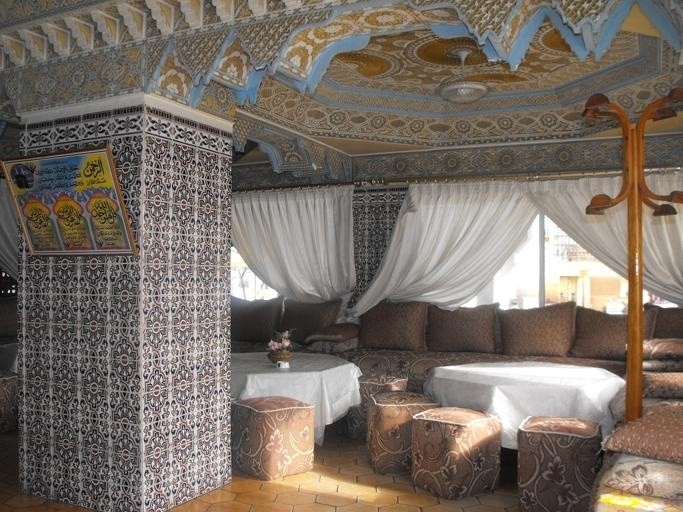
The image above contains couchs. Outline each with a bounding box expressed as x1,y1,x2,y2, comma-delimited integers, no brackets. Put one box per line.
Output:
595,370,681,512
231,322,358,358
352,348,682,423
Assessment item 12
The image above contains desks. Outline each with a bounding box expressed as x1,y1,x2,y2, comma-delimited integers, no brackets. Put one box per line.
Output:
231,350,363,447
433,360,627,466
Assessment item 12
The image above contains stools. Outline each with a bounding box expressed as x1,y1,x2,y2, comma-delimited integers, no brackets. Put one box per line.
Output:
410,406,503,499
230,395,316,482
366,390,443,475
0,370,16,436
518,413,605,511
344,371,407,443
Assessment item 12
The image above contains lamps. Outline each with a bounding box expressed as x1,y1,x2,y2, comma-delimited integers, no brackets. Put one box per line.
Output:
437,44,491,104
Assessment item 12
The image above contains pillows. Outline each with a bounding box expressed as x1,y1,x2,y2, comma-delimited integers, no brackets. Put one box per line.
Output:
625,303,683,359
428,302,502,354
570,303,659,363
231,294,344,343
500,300,579,356
357,301,429,353
594,402,682,502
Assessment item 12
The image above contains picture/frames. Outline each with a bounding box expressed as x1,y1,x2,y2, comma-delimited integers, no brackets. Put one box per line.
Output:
0,148,140,257
